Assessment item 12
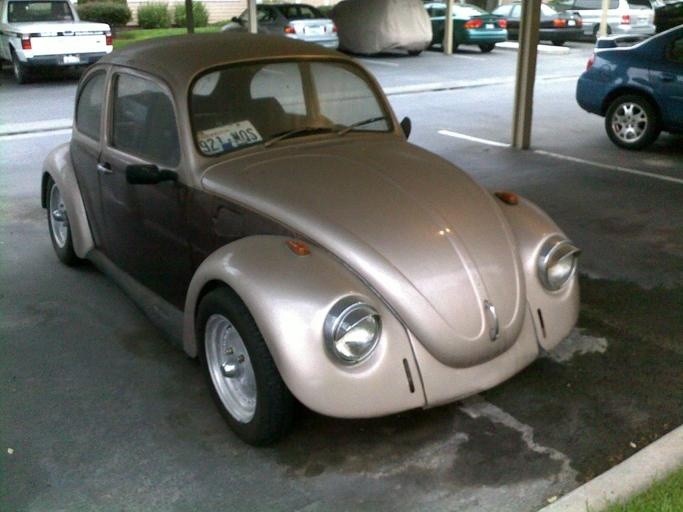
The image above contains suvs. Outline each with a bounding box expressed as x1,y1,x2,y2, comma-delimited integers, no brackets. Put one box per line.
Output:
561,0,656,45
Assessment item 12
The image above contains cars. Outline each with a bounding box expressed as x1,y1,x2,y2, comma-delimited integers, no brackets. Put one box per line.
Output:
593,31,650,49
491,2,584,46
423,2,511,54
218,4,340,53
39,29,583,450
654,1,682,34
574,25,682,149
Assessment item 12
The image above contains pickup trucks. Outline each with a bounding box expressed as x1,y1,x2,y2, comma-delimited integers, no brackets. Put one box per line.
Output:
0,0,116,86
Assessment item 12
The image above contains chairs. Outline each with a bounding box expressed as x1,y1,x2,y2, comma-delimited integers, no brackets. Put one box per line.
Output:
219,96,295,139
144,113,216,165
